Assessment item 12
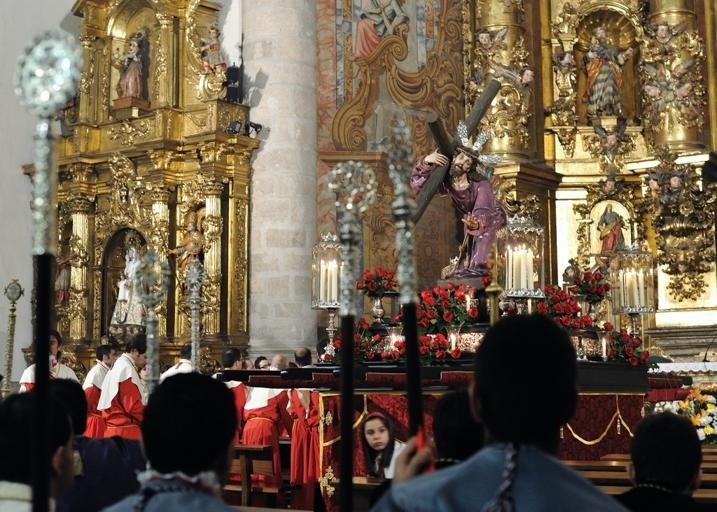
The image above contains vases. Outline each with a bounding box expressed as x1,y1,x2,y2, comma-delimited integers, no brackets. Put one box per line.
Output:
597,331,610,353
587,304,598,325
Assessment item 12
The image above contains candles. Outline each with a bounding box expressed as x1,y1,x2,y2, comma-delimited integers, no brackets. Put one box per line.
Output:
506,244,534,291
601,337,606,356
620,268,646,307
528,298,533,315
320,260,338,306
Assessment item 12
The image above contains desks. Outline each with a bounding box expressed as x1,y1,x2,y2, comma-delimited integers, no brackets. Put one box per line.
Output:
314,359,650,395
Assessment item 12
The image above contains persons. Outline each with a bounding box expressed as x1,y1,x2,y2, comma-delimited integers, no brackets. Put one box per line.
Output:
1,313,322,512
115,24,146,97
474,0,693,253
412,137,507,277
355,0,410,58
361,410,408,480
368,390,484,512
54,212,203,329
610,411,717,512
188,21,228,80
368,314,635,512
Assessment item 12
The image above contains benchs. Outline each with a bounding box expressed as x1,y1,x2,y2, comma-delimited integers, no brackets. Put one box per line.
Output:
328,477,383,512
231,444,272,506
562,449,716,504
702,447,717,453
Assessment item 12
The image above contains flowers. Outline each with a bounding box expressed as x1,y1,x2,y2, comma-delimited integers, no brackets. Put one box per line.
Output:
322,267,477,368
569,272,613,303
605,330,660,370
648,390,717,442
599,323,613,335
523,287,592,329
